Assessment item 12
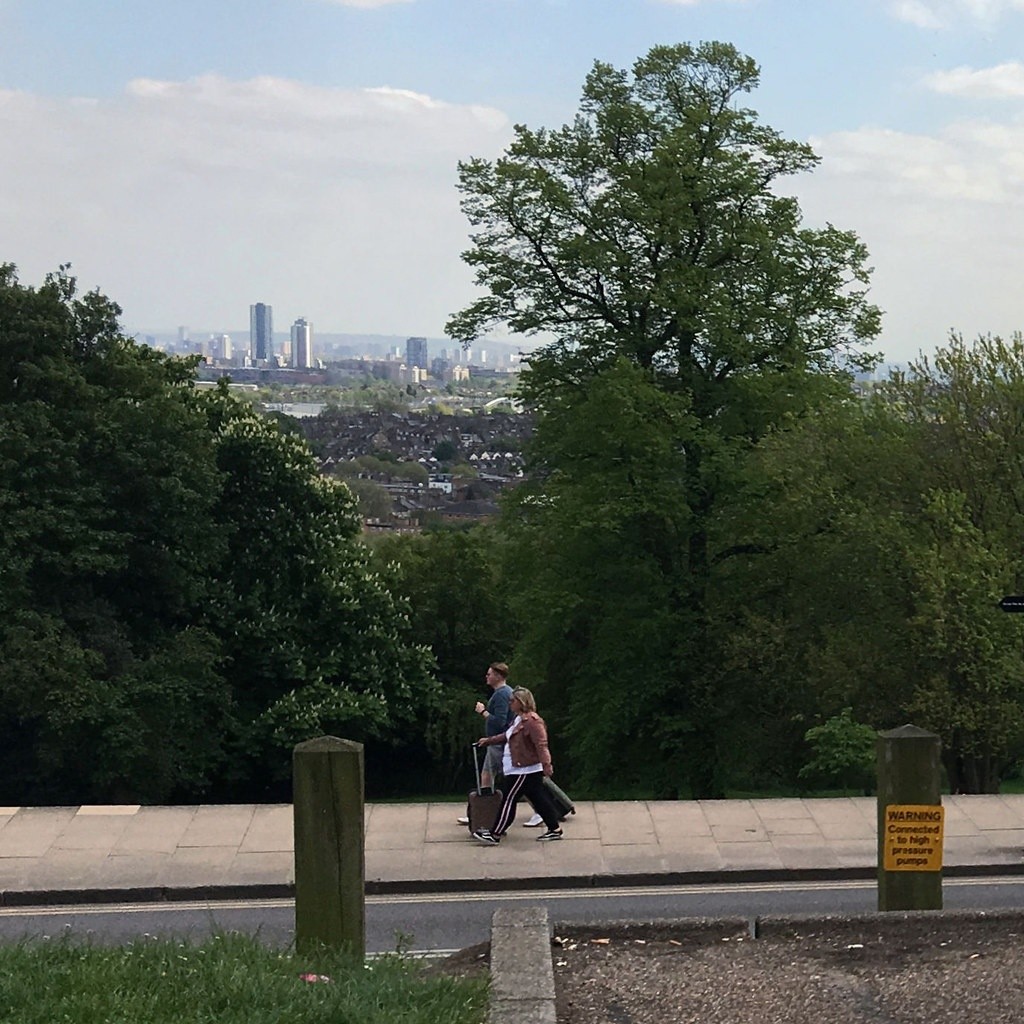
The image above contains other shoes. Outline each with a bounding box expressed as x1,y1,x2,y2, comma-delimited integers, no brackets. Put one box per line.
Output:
523,813,543,827
457,816,470,824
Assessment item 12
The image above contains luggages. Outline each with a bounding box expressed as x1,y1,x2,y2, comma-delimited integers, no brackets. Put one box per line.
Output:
467,741,505,835
543,776,576,822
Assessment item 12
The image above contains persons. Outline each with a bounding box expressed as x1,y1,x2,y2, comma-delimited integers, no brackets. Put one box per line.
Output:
457,661,545,826
473,685,564,846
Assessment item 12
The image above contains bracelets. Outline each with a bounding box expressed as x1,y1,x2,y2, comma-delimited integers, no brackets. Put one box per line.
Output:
480,710,486,715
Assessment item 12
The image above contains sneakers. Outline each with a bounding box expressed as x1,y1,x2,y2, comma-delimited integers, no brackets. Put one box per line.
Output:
473,830,501,845
537,829,564,840
